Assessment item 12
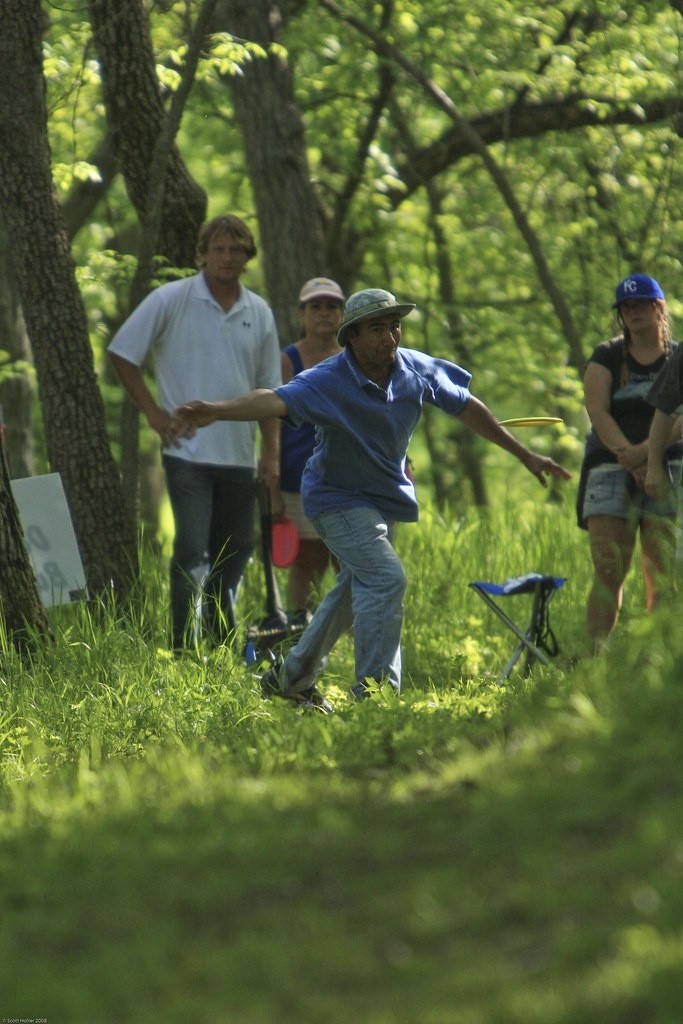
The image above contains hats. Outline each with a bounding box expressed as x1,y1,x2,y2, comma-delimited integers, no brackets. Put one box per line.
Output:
612,274,664,309
338,287,415,347
300,277,347,306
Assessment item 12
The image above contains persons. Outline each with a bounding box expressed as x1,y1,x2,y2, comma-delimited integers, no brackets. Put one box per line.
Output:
172,288,571,705
99,213,282,656
644,342,683,501
277,276,346,628
575,272,678,665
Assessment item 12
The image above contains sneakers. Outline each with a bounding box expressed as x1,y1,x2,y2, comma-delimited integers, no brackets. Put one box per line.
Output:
260,663,336,714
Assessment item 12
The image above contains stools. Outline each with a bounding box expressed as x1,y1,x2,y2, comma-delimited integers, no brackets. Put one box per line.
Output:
468,573,568,685
